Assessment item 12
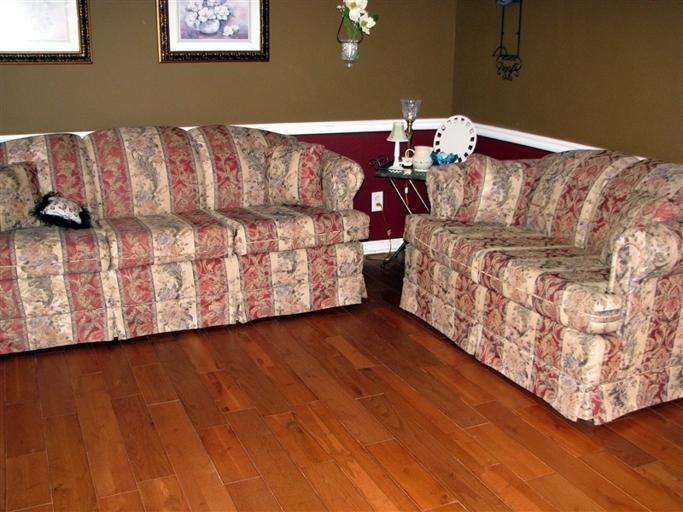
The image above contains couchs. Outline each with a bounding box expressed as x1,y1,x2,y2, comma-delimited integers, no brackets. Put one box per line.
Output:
399,149,683,427
0,124,370,359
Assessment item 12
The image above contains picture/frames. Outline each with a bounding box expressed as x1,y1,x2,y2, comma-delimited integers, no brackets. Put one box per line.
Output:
155,0,270,63
0,0,93,65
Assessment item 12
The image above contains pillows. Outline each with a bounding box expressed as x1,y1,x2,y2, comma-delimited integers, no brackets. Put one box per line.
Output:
0,161,92,232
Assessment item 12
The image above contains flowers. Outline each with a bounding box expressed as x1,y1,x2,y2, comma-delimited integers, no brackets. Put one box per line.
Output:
334,0,380,43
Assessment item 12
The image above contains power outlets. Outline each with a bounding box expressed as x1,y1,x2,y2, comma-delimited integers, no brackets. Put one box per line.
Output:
371,191,384,212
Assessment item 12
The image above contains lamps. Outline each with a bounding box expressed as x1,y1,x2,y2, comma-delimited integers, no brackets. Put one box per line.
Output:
386,122,409,173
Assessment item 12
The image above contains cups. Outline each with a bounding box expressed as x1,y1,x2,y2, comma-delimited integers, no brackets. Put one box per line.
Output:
404,145,434,173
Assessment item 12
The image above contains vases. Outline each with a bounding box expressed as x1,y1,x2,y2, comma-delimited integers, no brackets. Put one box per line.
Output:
340,40,359,69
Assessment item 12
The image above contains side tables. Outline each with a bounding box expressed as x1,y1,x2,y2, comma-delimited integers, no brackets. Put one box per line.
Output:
375,162,430,270
